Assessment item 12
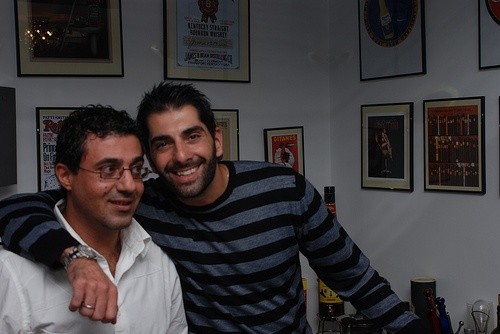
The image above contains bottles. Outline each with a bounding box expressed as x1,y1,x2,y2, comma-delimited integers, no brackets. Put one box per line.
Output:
318,185,345,318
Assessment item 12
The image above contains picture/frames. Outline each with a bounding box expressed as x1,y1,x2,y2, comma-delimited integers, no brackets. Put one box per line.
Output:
14,0,125,77
211,108,239,160
162,0,251,83
35,106,87,192
360,102,414,192
423,95,486,195
263,126,305,177
477,0,500,71
357,0,426,82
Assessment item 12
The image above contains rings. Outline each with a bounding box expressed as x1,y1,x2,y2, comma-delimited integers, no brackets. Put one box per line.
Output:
82,303,95,309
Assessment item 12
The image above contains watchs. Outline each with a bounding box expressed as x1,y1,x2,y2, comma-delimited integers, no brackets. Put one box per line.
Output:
63,245,98,269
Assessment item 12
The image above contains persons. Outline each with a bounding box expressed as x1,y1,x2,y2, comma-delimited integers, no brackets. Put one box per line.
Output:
0,103,189,334
0,77,431,334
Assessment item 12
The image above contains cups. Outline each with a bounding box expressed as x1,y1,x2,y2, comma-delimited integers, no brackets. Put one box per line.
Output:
410,278,437,308
